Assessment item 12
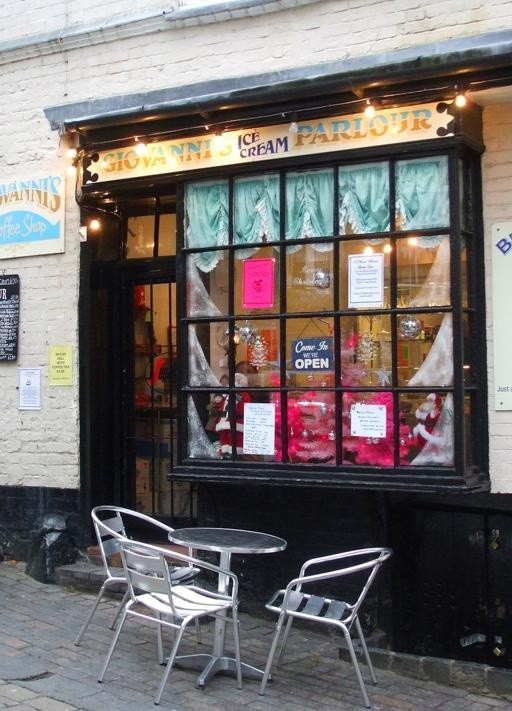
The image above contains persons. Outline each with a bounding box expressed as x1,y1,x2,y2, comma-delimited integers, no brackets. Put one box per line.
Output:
229,359,273,406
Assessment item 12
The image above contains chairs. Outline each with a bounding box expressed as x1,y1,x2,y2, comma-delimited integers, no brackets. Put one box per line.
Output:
97,538,243,705
258,547,394,708
75,506,201,665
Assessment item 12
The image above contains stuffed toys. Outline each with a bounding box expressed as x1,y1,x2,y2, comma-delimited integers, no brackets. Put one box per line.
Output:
398,393,443,455
212,371,254,459
204,394,225,441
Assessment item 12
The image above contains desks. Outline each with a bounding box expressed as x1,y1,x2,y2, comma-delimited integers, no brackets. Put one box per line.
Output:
164,527,287,689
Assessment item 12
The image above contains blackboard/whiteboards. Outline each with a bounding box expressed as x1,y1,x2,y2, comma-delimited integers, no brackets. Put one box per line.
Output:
0,274,19,361
